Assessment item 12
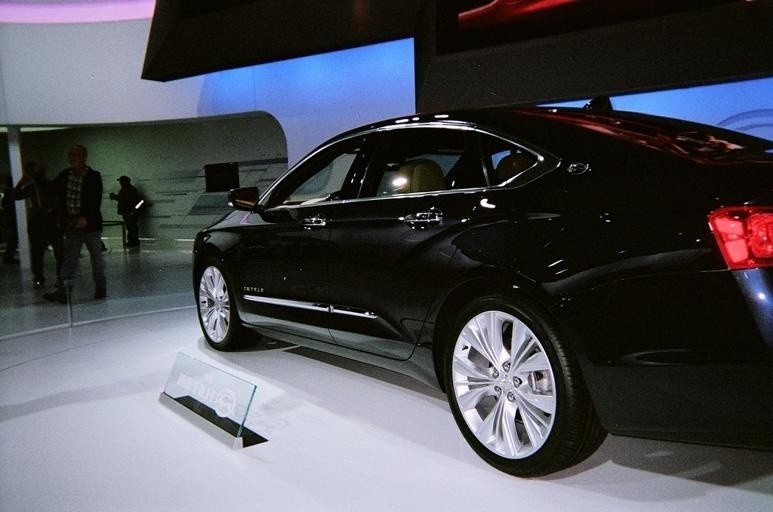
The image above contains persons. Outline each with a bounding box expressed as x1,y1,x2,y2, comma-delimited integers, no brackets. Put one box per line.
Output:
108,174,144,247
41,142,106,305
8,158,62,290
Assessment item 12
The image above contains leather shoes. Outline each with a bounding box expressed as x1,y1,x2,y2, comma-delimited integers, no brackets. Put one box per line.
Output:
126,242,140,247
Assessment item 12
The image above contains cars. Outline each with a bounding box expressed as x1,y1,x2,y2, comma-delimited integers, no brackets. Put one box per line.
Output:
188,95,773,479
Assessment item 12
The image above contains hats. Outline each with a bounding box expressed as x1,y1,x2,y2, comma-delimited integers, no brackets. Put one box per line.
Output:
117,176,131,181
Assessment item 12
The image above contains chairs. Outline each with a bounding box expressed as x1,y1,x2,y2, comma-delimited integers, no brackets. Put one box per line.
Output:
394,159,444,193
495,154,535,178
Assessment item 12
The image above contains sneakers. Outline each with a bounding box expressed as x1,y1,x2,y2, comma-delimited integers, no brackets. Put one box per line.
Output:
42,290,71,304
95,276,106,299
33,278,44,289
